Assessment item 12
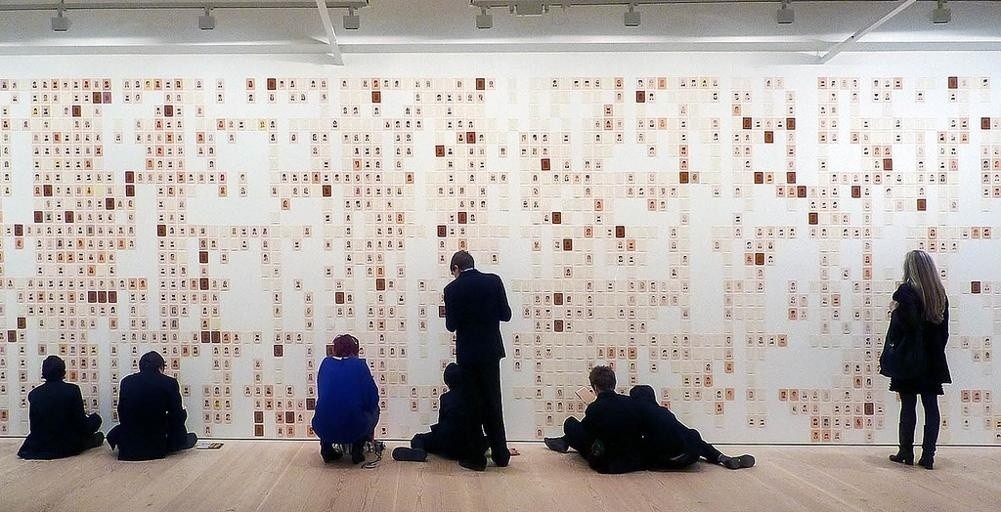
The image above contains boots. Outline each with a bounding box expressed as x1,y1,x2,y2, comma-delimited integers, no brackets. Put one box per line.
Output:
320,444,366,465
889,422,915,466
918,424,940,470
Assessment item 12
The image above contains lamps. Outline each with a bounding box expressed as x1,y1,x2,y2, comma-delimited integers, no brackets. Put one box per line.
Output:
469,0,956,27
50,0,365,32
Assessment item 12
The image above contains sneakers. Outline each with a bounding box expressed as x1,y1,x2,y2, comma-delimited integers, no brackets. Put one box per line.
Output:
724,454,755,470
544,436,570,452
391,445,428,461
457,457,486,471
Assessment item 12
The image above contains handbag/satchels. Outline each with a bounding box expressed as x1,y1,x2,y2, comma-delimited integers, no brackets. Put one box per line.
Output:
878,322,910,381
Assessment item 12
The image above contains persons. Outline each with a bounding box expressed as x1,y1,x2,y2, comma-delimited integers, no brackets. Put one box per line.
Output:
390,361,520,463
551,363,691,472
113,349,198,464
443,249,513,470
629,381,756,471
26,353,104,459
888,250,951,470
310,333,380,463
